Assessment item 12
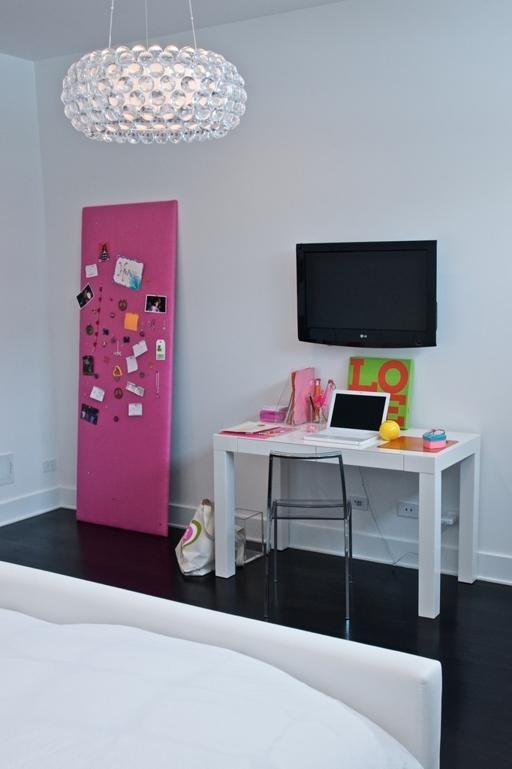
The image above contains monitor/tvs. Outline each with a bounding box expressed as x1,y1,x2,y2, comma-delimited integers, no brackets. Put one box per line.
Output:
296,242,437,350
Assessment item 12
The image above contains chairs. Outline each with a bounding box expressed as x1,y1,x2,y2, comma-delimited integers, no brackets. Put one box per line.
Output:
262,449,354,636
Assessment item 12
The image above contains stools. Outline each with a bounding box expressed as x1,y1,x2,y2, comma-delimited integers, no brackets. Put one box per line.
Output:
233,508,265,565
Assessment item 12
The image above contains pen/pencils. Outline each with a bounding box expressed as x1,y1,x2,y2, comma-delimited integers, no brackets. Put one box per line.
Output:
309,379,336,424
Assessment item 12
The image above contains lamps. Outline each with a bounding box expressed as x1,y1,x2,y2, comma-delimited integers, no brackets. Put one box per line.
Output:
59,1,249,146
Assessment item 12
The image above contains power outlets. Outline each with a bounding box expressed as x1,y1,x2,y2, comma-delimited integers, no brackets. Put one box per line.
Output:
442,511,458,525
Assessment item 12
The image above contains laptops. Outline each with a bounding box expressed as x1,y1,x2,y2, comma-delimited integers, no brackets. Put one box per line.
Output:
302,390,391,446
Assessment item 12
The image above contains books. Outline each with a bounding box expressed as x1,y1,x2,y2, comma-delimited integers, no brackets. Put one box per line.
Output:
290,367,315,425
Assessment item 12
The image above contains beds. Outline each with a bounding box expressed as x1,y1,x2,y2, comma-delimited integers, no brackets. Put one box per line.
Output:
0,559,445,769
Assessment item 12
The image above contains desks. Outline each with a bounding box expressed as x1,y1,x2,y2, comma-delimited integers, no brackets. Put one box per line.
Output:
211,423,482,618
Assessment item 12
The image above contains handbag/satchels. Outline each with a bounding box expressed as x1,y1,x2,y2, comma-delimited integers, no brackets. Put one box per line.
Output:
174,497,245,576
